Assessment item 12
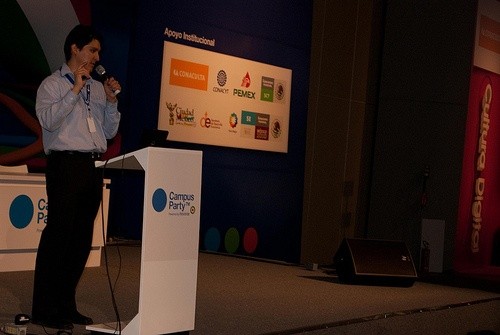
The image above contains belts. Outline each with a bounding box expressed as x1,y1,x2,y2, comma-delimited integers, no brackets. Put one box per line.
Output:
53,150,101,159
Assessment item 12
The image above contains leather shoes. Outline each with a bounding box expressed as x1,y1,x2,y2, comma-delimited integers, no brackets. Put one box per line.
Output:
31,321,75,329
71,313,93,325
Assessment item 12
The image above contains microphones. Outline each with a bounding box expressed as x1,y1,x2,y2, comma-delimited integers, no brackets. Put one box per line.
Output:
96,65,120,96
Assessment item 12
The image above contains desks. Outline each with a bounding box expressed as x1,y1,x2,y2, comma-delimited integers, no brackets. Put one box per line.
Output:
0,172,111,273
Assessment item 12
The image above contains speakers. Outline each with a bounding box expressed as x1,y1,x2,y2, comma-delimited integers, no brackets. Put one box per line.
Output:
333,236,418,287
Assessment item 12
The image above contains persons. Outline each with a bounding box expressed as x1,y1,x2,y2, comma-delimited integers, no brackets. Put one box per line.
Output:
28,23,121,330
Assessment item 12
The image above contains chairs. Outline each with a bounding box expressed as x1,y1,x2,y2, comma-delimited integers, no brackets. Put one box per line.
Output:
0,164,29,173
